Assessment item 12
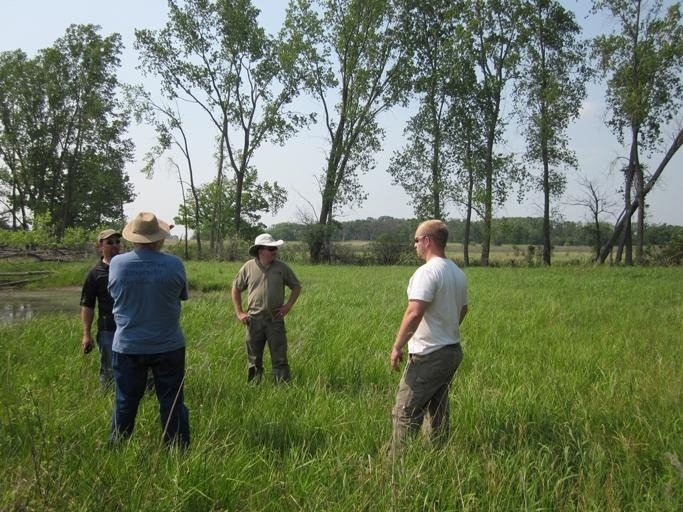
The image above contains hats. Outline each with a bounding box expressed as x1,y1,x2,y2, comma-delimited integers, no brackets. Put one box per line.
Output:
97,229,122,244
122,212,171,244
248,232,285,257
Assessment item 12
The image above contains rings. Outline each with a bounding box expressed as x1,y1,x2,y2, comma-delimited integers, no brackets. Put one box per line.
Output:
281,312,283,316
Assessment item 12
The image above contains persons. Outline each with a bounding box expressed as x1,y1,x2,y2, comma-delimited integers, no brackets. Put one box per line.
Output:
392,219,468,440
79,229,157,390
105,213,189,453
230,233,301,385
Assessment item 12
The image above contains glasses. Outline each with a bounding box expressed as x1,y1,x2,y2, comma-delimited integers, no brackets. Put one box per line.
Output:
106,239,120,245
261,246,278,251
